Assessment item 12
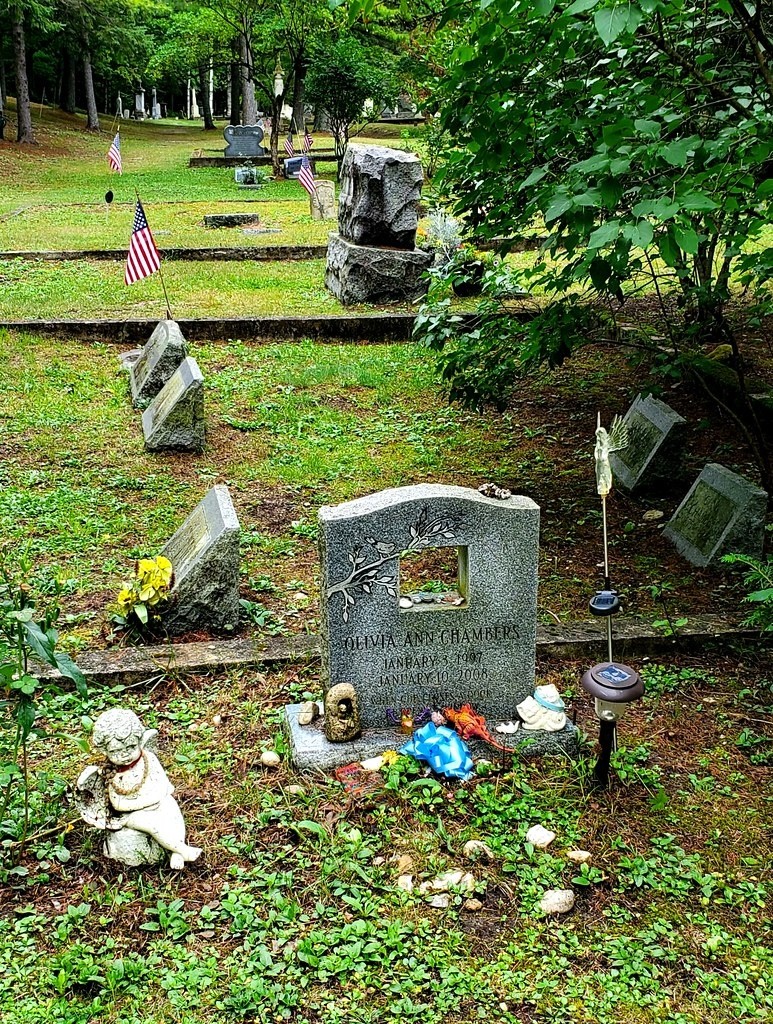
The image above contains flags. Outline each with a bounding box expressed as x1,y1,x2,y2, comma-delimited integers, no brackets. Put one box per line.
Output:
303,126,313,152
298,151,316,195
283,133,292,158
107,131,121,171
124,201,160,286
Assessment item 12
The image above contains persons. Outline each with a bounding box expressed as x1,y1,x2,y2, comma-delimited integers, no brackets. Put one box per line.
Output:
91,708,203,869
